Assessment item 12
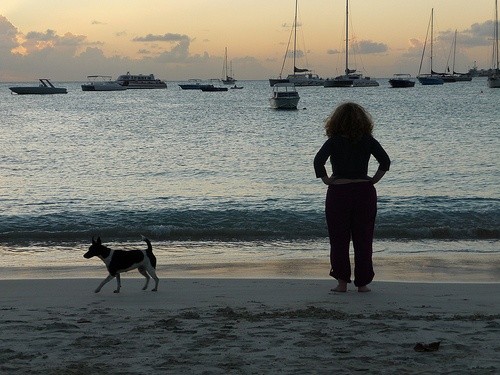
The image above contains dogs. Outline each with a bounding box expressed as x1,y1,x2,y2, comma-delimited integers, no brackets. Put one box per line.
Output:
83,233,159,294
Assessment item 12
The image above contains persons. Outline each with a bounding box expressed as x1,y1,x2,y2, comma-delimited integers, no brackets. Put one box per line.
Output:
313,102,391,293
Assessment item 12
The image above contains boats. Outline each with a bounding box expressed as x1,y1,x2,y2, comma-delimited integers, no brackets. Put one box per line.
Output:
230,85,243,89
178,82,214,89
81,76,127,91
9,79,68,94
116,72,167,89
200,87,228,91
270,87,300,110
388,74,416,88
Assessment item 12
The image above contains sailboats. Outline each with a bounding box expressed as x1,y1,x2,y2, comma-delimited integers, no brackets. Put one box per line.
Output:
324,0,379,87
269,0,326,87
221,47,237,84
416,8,473,84
487,0,500,88
